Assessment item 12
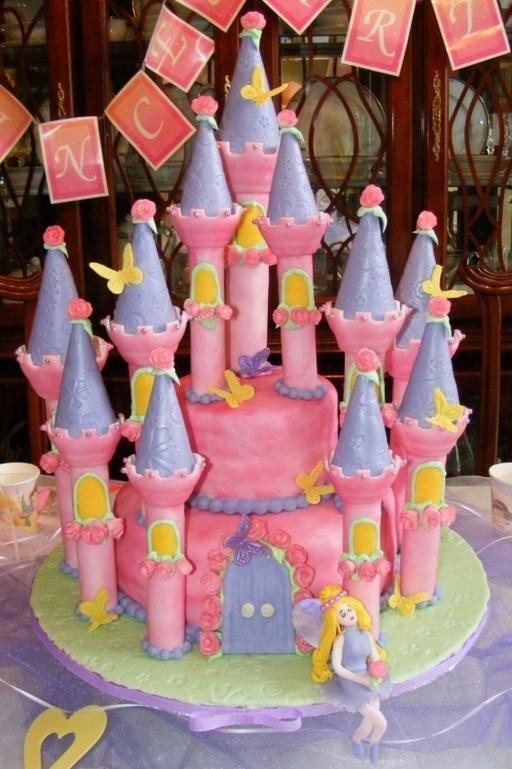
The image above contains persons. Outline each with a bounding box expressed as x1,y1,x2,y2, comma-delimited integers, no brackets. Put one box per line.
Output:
310,584,389,765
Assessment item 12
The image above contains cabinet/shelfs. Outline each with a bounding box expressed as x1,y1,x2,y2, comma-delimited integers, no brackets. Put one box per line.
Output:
0,0,512,349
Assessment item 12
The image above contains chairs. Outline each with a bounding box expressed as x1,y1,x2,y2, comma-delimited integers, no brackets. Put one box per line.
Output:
1,267,56,475
450,248,512,481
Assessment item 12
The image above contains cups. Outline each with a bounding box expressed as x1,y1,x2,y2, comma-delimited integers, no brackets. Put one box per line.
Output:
0,459,39,540
489,461,511,536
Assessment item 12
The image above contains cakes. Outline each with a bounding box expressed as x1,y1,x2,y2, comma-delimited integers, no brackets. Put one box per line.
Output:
15,8,491,763
